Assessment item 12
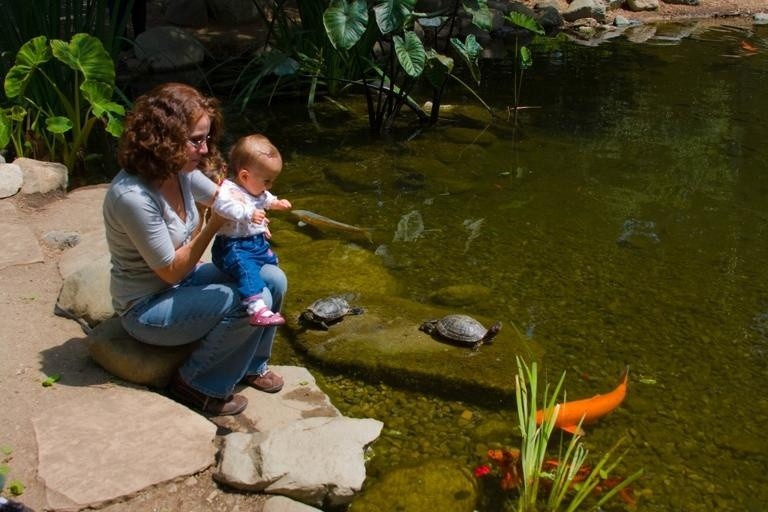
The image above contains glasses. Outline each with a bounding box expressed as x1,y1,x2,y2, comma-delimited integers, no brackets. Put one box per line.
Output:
184,135,212,149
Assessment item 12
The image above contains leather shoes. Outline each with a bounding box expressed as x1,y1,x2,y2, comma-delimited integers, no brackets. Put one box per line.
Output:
247,306,286,327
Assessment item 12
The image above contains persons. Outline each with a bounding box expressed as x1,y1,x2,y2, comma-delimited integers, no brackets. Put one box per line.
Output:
210,134,292,326
103,83,288,416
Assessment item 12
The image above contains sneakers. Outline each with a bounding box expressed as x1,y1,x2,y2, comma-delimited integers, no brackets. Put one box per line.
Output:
240,369,284,393
165,372,248,415
